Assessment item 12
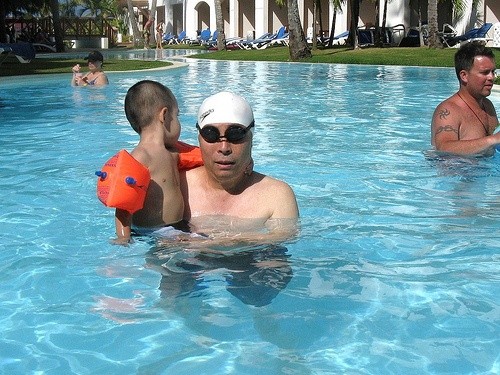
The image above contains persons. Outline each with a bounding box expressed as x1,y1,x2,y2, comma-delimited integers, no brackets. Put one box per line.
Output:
181,92,299,218
430,42,500,158
96,81,255,244
33,29,55,48
71,51,109,86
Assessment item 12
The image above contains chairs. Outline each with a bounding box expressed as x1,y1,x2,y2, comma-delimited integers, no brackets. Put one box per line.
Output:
162,23,500,51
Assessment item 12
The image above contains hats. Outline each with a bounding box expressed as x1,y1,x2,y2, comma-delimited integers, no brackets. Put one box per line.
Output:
84,52,103,62
196,92,255,134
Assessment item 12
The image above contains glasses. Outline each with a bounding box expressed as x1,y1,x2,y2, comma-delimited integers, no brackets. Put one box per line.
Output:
198,121,253,143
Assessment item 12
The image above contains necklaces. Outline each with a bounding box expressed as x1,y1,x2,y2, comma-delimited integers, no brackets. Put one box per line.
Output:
457,92,489,137
